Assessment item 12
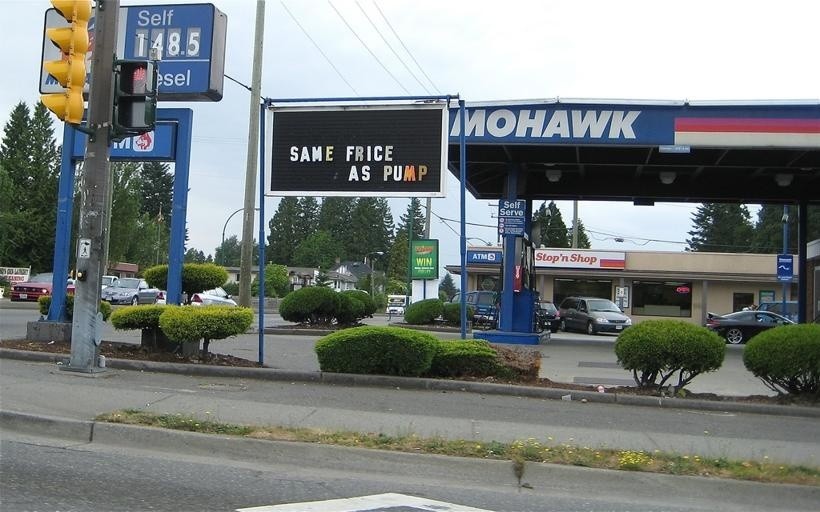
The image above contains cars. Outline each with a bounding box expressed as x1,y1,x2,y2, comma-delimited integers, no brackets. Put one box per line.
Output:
704,310,798,345
9,272,76,302
154,285,239,306
536,300,561,333
101,275,118,289
101,277,162,307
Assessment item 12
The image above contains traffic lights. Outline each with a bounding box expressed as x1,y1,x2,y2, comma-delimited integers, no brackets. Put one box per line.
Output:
106,55,158,139
39,0,93,128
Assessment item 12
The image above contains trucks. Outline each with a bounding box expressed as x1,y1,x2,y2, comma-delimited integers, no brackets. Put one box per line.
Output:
386,294,412,316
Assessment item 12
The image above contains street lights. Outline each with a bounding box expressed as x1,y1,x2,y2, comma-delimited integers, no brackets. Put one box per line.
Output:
466,235,491,246
363,251,384,265
221,207,261,266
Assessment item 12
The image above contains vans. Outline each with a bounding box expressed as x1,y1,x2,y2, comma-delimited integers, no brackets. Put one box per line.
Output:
558,296,633,336
449,291,499,322
758,301,800,327
